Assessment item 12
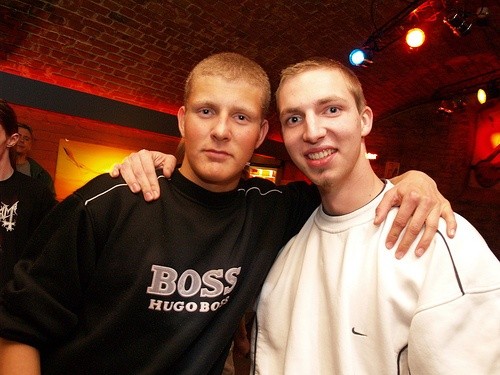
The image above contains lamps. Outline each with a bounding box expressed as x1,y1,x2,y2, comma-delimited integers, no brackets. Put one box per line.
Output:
443,10,489,37
349,44,376,68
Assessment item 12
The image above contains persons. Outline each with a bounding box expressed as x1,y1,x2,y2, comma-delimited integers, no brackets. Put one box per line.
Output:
110,57,500,375
15,124,54,191
0,103,56,278
0,52,457,375
